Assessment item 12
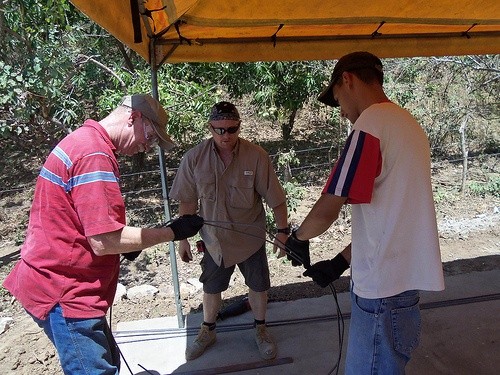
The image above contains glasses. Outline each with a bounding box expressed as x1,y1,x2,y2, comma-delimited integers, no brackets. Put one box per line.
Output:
209,123,239,135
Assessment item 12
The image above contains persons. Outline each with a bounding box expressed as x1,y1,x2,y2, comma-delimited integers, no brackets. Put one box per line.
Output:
167,101,288,359
284,52,446,375
4,93,171,375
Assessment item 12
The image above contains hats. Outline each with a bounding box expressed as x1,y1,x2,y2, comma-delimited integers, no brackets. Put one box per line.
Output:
122,94,175,150
317,52,383,107
211,102,240,120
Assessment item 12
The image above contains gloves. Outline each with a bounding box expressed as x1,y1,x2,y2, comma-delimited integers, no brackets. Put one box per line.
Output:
285,230,310,269
122,250,141,261
166,214,204,242
303,253,350,287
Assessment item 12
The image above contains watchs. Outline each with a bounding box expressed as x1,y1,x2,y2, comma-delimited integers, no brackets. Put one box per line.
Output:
278,227,290,235
291,227,309,244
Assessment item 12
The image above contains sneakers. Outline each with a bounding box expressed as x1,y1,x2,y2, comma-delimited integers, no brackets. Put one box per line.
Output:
185,323,216,361
255,326,275,360
